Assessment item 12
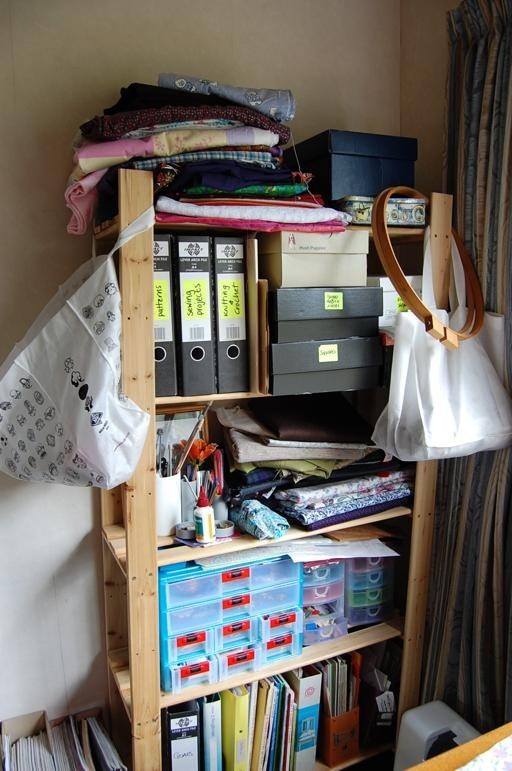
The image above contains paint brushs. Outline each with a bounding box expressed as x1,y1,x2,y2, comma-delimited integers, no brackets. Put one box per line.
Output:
172,415,204,476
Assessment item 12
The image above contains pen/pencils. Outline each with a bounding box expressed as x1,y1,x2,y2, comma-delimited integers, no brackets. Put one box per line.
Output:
163,684,249,771
169,444,172,476
183,458,219,505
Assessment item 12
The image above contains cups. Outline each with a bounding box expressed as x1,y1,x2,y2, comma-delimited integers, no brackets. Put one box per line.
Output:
182,478,196,522
156,471,183,535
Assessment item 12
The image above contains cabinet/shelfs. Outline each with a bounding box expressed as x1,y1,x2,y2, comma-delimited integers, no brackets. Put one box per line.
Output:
88,167,454,770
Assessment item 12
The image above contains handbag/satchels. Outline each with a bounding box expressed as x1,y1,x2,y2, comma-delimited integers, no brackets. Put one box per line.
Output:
0,209,151,490
371,225,512,462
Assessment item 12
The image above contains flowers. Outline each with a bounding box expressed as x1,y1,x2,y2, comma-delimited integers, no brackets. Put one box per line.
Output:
180,437,217,472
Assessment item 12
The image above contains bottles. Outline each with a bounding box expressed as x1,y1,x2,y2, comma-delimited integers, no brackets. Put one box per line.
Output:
213,493,229,522
195,488,215,545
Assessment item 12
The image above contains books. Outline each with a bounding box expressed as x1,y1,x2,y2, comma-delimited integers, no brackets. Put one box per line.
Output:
1,714,128,771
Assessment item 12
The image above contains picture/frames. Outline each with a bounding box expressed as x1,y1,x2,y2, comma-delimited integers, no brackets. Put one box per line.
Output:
155,403,211,449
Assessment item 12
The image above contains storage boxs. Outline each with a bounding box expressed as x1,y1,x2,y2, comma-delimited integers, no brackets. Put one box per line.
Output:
259,226,421,398
285,130,418,202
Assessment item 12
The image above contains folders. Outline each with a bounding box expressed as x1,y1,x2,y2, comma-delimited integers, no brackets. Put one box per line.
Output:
153,235,250,397
